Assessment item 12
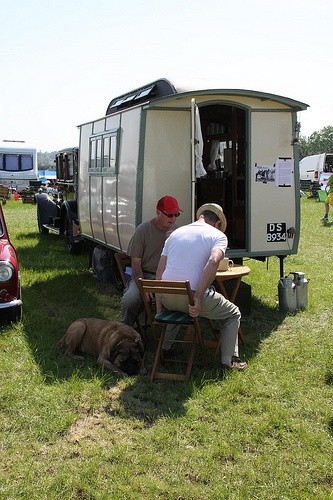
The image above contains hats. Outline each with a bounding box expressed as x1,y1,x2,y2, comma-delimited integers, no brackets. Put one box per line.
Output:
157,196,183,214
196,203,227,233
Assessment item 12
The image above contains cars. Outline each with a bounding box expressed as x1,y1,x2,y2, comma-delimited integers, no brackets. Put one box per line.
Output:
34,146,82,253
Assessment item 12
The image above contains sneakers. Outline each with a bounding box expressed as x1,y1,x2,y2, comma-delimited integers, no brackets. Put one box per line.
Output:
223,356,247,370
162,345,184,358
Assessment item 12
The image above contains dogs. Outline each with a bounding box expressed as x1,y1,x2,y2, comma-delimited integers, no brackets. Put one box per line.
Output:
55,317,148,378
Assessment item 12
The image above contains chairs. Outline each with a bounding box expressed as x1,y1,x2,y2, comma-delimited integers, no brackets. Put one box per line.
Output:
138,277,205,384
115,252,157,344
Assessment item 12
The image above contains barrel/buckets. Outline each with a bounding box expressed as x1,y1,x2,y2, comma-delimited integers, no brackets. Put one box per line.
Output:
277,276,297,311
288,272,308,310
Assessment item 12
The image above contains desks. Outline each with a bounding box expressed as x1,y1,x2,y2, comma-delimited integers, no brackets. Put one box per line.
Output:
204,264,251,356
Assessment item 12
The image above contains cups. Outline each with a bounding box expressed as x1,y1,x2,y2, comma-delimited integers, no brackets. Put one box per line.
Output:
219,257,233,271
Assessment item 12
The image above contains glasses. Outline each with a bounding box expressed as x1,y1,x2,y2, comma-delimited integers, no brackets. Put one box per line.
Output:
160,210,180,218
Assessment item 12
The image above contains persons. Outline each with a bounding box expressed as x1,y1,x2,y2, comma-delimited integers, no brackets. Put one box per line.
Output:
121,195,183,332
320,164,333,223
155,203,247,372
